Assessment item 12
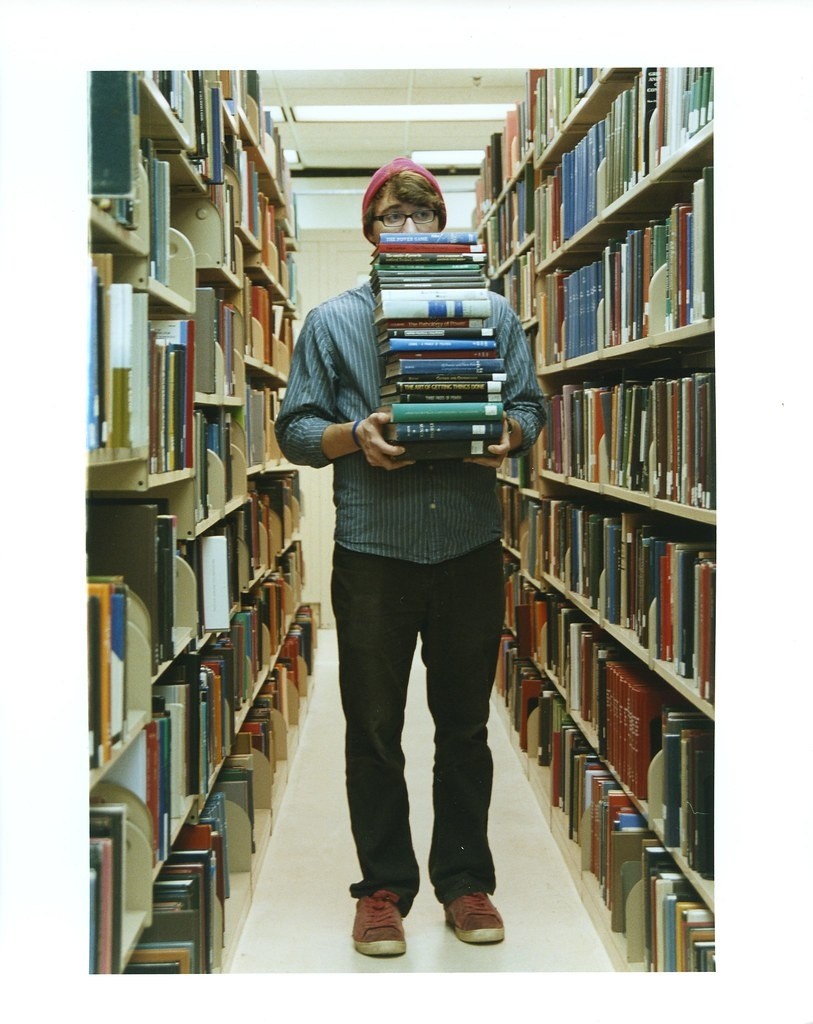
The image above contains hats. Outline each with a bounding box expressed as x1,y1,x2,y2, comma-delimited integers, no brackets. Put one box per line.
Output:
362,158,447,247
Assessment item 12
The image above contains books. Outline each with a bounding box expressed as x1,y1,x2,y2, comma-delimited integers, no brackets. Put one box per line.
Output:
511,67,716,970
87,72,317,971
474,132,512,707
370,231,509,462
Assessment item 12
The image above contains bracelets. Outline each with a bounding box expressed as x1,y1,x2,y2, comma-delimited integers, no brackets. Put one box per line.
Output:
351,421,361,448
506,418,514,434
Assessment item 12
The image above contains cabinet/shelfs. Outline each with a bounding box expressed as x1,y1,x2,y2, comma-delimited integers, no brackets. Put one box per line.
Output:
472,68,714,973
89,72,326,975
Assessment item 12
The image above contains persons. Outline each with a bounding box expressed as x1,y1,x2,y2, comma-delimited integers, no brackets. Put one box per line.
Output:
274,159,550,960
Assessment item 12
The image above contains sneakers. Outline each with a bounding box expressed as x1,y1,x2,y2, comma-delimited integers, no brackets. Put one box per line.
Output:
445,891,504,943
353,891,406,956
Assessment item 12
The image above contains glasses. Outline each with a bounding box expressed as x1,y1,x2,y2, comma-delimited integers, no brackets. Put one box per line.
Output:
372,210,440,227
